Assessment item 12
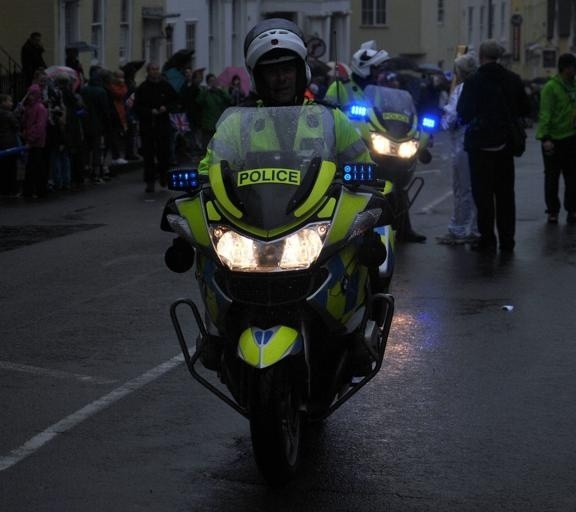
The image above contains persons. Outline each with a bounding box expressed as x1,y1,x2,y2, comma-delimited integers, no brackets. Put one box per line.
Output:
198,18,384,378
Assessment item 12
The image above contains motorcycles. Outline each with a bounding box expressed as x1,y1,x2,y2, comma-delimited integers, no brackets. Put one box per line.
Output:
348,84,437,241
162,102,405,482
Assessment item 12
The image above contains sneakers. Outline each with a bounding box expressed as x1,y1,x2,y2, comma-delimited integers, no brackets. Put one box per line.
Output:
434,233,479,245
547,213,558,223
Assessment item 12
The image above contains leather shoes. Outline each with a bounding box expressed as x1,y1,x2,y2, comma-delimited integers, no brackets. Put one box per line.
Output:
395,229,427,244
145,176,167,193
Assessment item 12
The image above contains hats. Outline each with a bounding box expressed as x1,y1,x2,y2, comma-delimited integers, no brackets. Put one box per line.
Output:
456,54,474,74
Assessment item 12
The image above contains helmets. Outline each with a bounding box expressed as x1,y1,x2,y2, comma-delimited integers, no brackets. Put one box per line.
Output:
351,48,390,86
245,19,308,105
